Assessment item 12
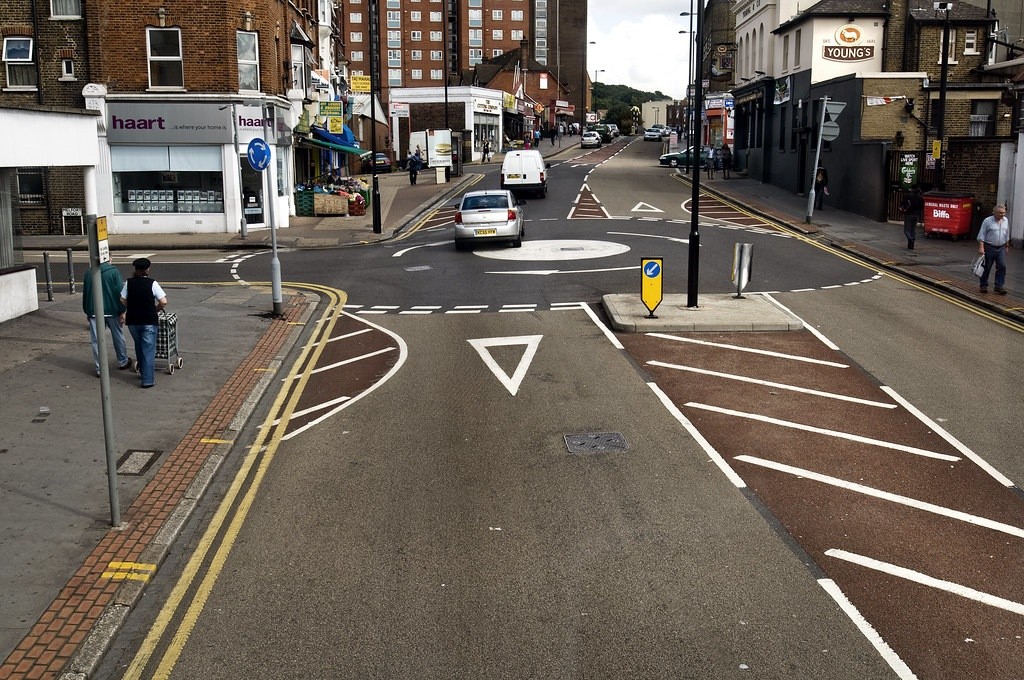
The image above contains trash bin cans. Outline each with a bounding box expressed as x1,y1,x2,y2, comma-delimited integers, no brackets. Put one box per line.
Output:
922,191,976,235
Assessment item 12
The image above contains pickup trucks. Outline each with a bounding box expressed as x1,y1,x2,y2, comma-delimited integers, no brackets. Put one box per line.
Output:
666,126,672,136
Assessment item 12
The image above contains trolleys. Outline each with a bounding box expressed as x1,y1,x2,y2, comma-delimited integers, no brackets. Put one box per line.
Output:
135,309,183,376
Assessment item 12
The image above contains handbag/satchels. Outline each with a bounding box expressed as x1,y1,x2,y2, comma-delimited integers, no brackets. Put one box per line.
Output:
487,152,492,159
703,162,708,172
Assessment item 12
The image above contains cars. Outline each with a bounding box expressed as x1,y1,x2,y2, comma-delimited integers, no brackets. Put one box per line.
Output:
659,145,723,167
454,189,525,250
671,128,677,134
361,153,392,174
607,124,620,137
581,131,603,148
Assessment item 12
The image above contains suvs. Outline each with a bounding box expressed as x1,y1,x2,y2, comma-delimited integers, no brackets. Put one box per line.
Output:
652,124,666,137
591,125,612,143
643,128,662,142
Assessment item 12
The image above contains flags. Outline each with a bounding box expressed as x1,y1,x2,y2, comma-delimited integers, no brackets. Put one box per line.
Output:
866,95,906,107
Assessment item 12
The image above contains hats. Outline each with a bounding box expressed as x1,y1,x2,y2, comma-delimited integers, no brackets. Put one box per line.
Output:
133,258,151,270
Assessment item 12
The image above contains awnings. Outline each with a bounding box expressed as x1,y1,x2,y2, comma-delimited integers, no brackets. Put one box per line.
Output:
311,127,360,150
326,123,354,143
301,136,372,169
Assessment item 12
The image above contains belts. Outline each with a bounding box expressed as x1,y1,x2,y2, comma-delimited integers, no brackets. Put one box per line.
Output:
985,242,1005,249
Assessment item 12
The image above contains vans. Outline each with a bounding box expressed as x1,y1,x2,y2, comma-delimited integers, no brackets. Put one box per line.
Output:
500,149,556,197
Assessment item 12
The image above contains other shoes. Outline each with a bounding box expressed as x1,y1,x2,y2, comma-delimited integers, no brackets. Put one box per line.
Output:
141,384,154,388
908,238,915,250
980,287,988,293
119,358,132,370
96,370,100,378
993,287,1007,295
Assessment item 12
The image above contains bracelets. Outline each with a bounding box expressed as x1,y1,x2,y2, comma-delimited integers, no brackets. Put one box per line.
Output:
1005,245,1009,248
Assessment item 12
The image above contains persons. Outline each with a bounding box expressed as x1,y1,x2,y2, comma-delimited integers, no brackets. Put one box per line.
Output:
481,139,491,162
120,258,168,388
327,169,344,186
524,135,533,150
406,145,424,186
811,159,828,210
977,204,1010,295
900,184,924,249
720,144,733,179
548,126,559,145
676,122,683,144
82,253,133,378
558,123,584,139
534,128,541,148
705,144,717,180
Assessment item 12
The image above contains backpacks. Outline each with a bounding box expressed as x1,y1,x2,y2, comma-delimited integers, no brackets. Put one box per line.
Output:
409,156,418,168
969,250,986,279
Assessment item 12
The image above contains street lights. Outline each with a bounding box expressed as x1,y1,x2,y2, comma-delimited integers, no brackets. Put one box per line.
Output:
595,69,605,112
580,41,597,134
218,102,249,240
678,29,696,144
652,106,659,124
